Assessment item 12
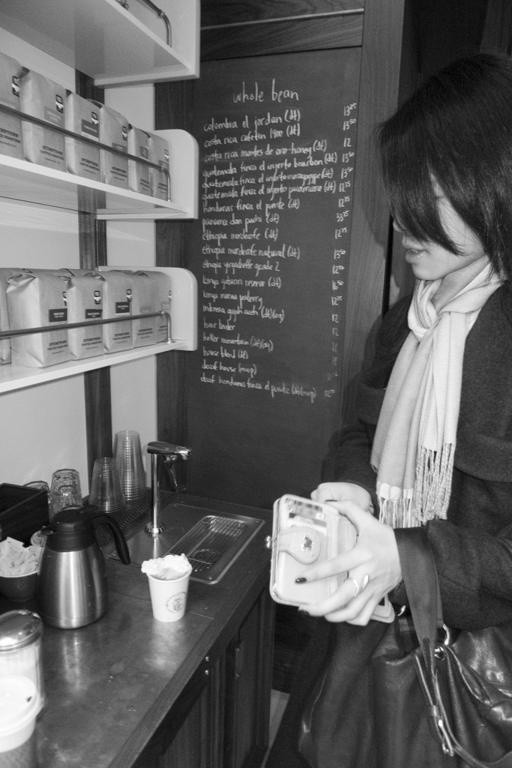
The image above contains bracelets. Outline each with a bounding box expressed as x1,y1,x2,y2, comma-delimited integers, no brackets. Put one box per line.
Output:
1,572,38,605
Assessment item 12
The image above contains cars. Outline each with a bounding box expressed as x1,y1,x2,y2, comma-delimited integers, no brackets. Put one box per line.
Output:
147,566,191,624
24,430,147,518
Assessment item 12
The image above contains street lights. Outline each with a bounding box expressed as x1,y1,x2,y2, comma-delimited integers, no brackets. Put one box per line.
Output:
297,602,511,768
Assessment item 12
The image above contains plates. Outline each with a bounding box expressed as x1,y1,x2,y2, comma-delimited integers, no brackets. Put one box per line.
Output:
148,581,277,768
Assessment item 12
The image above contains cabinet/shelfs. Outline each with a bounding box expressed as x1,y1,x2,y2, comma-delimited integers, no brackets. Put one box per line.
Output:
148,581,277,768
2,58,198,220
3,265,198,392
4,1,200,87
0,608,46,733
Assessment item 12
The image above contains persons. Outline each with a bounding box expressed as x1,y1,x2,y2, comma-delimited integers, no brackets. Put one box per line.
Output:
264,49,511,765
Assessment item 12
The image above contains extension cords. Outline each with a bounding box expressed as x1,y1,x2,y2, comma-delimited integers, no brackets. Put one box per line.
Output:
40,503,133,631
